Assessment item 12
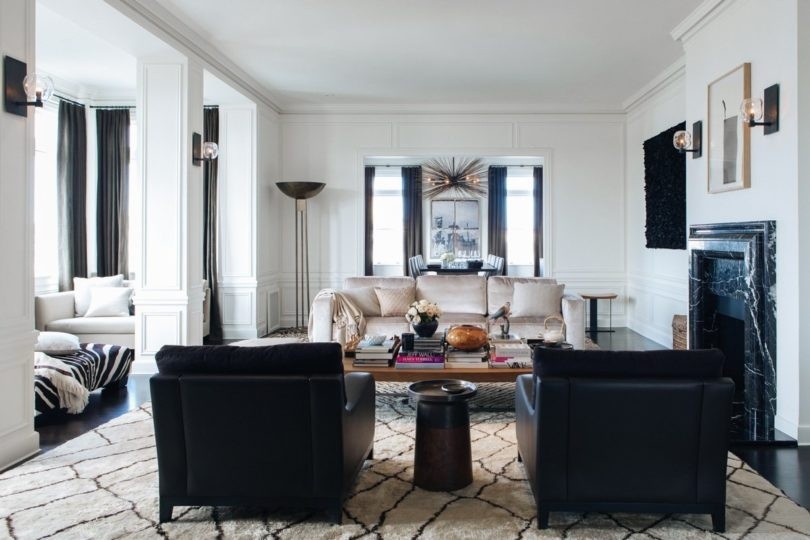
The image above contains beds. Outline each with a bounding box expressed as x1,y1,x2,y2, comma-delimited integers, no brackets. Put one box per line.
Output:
33,343,134,423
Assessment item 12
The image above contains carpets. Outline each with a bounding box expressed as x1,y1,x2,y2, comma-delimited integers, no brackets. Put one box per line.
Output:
0,329,810,539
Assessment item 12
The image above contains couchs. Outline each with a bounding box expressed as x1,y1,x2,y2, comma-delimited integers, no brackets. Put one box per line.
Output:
515,349,735,532
311,274,585,350
150,343,375,524
35,280,212,348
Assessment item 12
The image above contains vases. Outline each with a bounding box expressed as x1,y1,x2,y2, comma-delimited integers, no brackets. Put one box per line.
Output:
414,317,438,336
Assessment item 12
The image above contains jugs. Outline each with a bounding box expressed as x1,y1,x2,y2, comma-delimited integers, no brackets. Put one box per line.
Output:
540,315,565,347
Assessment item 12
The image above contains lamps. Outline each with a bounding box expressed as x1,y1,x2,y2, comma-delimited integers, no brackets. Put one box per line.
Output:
740,84,780,135
4,56,55,118
192,132,219,166
415,152,491,261
672,121,701,159
274,181,325,336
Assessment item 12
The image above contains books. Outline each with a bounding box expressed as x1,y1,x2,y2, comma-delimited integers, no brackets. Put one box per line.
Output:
488,334,534,369
444,345,488,369
395,332,446,368
353,333,399,367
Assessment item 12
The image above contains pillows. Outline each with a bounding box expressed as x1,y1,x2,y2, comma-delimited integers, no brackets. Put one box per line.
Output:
34,329,83,357
74,273,133,316
510,284,564,318
338,286,417,319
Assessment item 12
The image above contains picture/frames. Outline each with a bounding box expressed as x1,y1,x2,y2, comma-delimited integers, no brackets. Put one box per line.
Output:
707,62,752,196
430,199,481,259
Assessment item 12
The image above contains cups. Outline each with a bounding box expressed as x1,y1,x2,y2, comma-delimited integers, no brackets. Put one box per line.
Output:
402,333,414,352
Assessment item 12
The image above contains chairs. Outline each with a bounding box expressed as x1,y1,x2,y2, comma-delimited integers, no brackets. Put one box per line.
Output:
407,251,504,277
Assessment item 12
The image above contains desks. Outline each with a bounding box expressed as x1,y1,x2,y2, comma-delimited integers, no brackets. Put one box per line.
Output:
580,292,617,334
419,261,497,275
408,379,479,490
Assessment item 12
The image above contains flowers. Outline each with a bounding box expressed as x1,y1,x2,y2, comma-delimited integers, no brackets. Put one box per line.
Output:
404,298,441,325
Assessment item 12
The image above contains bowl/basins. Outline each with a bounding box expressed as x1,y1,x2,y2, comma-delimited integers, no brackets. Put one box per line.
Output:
365,333,386,345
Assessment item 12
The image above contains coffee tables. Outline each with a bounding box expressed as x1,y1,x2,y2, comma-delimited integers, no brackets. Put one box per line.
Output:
338,339,535,382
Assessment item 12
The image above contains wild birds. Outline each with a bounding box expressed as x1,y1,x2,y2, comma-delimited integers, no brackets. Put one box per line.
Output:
489,301,511,321
